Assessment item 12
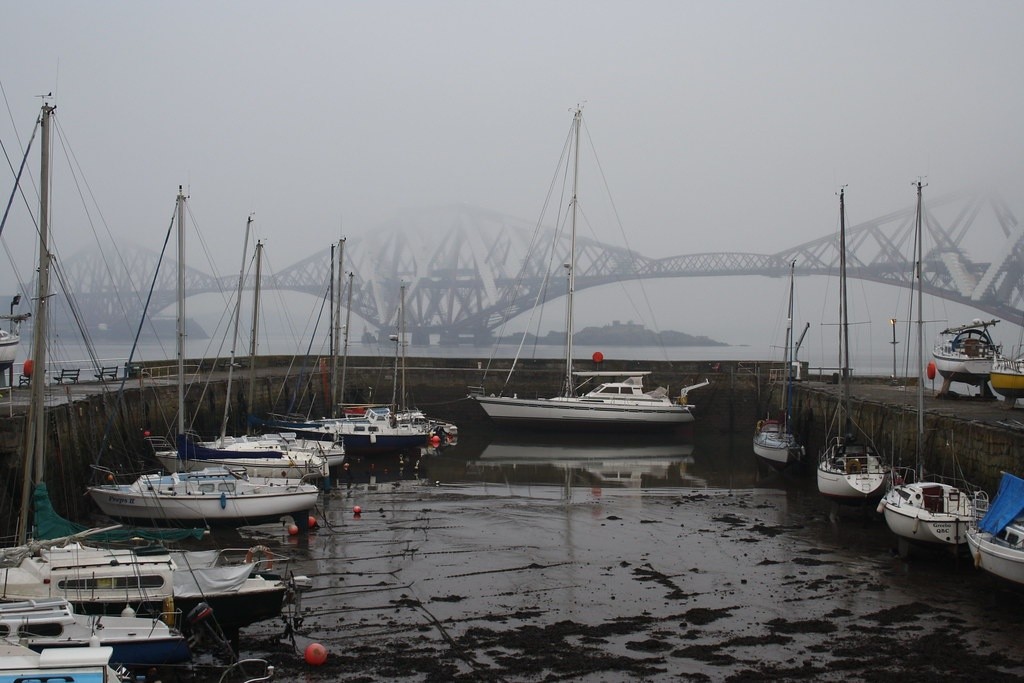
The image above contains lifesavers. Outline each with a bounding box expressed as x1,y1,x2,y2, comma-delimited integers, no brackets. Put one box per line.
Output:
593,352,603,363
757,420,765,429
247,544,272,572
846,459,861,473
681,396,687,403
163,596,174,625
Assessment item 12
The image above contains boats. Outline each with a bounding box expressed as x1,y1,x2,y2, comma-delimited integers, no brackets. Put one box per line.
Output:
931,318,1004,385
0,569,192,666
361,325,377,343
965,471,1024,584
988,356,1024,408
0,636,140,683
0,295,21,362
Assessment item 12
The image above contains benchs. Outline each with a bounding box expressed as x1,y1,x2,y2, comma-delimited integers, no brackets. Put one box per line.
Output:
240,359,256,371
218,359,234,372
53,368,81,385
18,368,48,389
93,366,120,382
194,360,215,373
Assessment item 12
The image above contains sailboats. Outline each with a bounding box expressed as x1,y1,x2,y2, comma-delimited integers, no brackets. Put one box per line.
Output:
468,103,710,433
876,174,990,545
817,188,885,499
197,238,346,466
245,239,458,452
752,259,805,466
88,184,320,520
0,103,314,629
144,217,330,480
